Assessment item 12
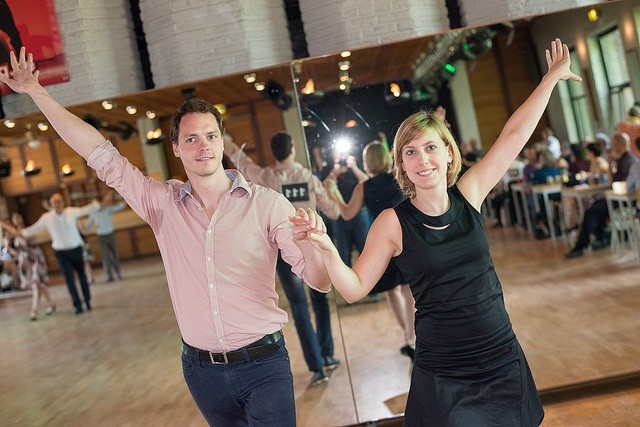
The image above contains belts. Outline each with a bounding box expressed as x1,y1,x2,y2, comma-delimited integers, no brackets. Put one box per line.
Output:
181,331,281,365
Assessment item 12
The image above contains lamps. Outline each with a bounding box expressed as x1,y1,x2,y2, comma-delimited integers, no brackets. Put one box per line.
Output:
265,79,290,110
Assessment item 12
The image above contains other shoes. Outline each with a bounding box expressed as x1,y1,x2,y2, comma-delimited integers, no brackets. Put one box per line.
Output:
323,356,341,369
75,303,82,313
593,240,610,248
312,371,328,385
31,310,36,320
567,249,583,257
45,305,55,314
401,345,416,361
85,300,91,309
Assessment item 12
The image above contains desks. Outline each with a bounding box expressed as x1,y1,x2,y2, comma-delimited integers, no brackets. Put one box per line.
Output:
508,170,640,264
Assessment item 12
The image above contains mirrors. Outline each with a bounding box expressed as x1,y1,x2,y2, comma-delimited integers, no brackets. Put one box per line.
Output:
0,0,640,427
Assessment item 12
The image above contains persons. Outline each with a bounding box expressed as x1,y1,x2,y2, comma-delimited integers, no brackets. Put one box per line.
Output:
223,129,341,386
488,127,562,228
313,145,380,300
290,38,582,427
459,135,492,218
0,211,56,320
42,200,96,284
15,190,113,315
83,195,128,281
557,132,640,259
324,106,450,348
0,46,333,427
615,100,640,159
